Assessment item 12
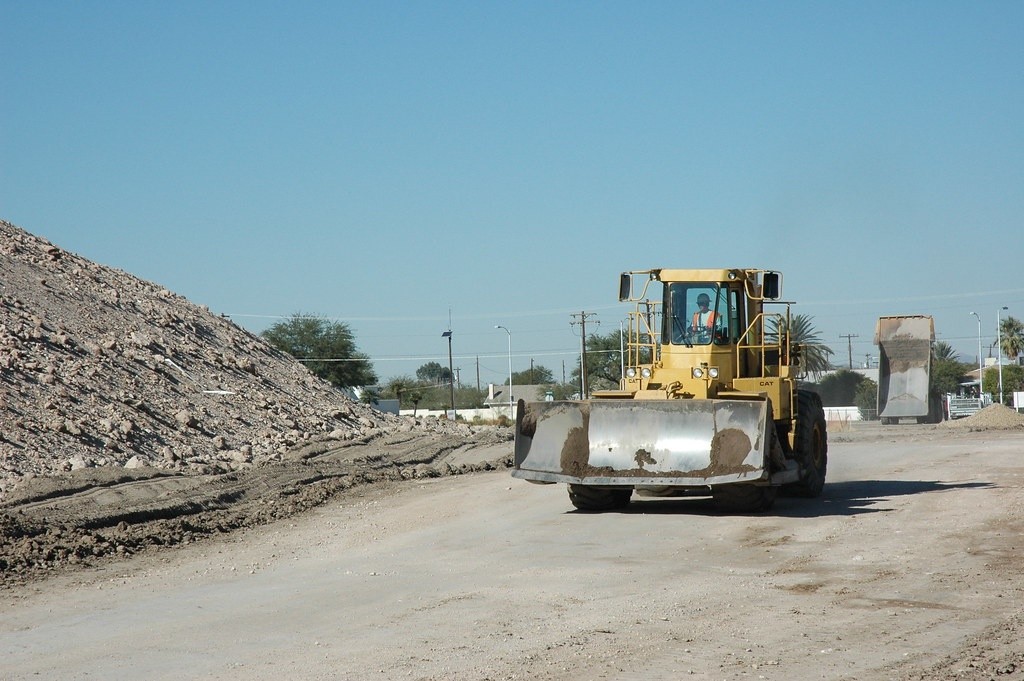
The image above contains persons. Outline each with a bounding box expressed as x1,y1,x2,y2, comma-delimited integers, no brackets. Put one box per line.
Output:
680,293,722,344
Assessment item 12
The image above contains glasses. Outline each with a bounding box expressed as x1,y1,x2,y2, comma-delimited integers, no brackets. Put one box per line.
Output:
697,303,705,307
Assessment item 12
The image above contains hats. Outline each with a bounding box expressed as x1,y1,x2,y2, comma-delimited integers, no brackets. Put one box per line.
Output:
696,293,712,303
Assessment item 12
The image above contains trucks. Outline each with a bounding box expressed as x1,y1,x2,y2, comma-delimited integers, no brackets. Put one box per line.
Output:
874,314,942,425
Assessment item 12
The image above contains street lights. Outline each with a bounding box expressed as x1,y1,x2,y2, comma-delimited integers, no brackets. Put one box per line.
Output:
495,325,513,419
998,306,1008,403
442,330,454,410
970,312,983,400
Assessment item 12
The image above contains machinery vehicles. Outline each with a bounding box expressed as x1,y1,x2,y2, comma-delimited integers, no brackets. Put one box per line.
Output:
511,267,828,515
